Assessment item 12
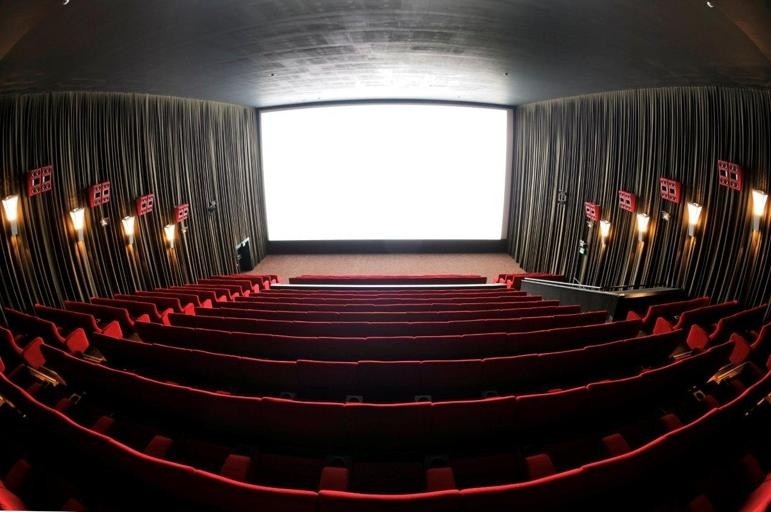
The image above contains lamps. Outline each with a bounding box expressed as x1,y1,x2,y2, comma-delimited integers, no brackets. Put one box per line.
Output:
688,202,703,236
1,194,22,235
752,189,768,231
164,224,176,248
122,216,135,244
69,207,85,241
636,213,651,241
599,219,611,247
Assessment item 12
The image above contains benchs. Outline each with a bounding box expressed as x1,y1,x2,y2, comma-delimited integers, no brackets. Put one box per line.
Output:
0,272,771,511
